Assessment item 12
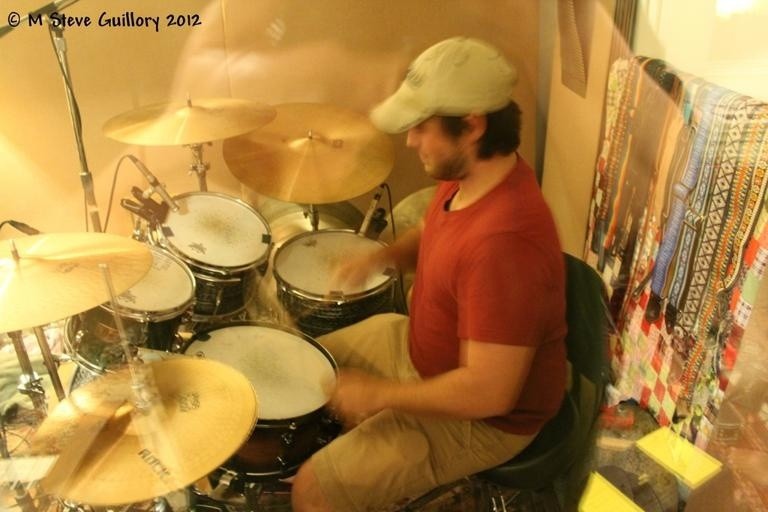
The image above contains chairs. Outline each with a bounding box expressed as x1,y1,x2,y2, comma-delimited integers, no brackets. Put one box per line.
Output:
404,252,609,512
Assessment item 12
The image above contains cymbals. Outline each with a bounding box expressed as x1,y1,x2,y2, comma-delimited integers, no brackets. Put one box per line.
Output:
223,102,396,204
27,357,258,507
0,230,153,334
102,96,277,146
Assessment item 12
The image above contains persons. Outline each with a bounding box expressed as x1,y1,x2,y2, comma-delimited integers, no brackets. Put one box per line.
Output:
289,35,569,511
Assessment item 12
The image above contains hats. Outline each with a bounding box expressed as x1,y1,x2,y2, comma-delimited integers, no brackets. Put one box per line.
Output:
370,38,512,133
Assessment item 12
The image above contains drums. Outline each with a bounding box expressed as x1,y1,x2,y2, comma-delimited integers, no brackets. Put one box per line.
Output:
274,227,397,337
147,191,273,323
61,241,197,376
177,320,340,482
